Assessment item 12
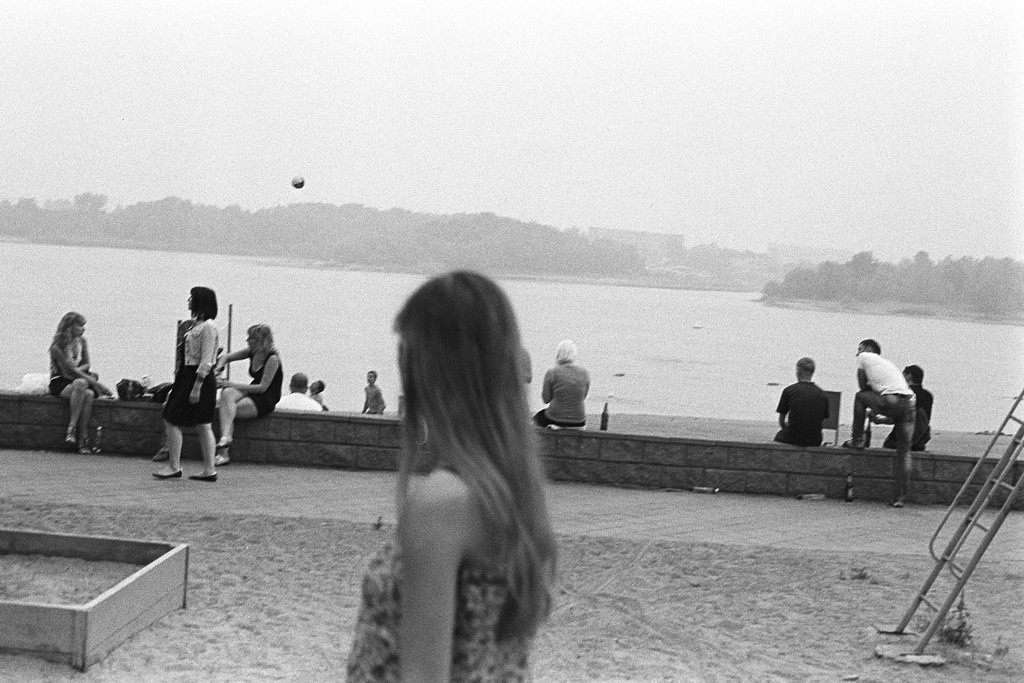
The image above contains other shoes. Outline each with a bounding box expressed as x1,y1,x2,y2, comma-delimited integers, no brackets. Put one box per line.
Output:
890,500,904,508
212,456,231,466
217,437,233,449
844,440,866,449
189,475,218,482
152,470,182,478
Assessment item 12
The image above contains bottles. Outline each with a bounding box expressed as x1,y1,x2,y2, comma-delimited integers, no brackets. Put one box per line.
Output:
846,470,854,502
863,420,872,448
600,402,609,431
126,381,134,401
92,425,102,454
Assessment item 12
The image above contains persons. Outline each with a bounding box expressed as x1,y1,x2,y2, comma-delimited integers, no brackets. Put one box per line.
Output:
309,380,325,404
346,271,558,683
361,370,386,413
882,365,933,450
276,371,323,411
215,324,283,467
774,357,830,447
520,344,532,383
48,311,112,453
152,320,194,461
151,286,218,482
533,338,590,428
842,339,916,507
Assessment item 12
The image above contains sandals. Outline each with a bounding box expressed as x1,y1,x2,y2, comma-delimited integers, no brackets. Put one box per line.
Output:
64,425,77,446
77,445,93,456
